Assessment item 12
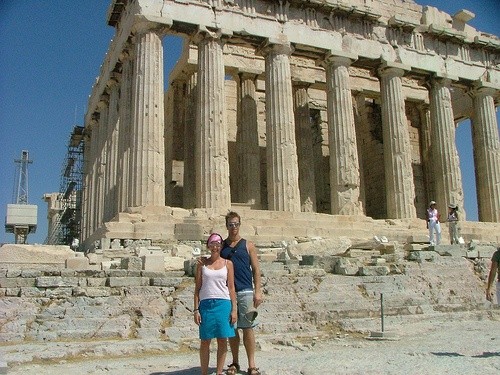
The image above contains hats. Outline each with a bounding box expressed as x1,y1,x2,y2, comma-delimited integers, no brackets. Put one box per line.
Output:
449,203,457,208
208,235,221,243
430,201,436,206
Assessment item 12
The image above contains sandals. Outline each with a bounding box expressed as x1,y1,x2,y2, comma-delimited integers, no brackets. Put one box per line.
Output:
226,363,239,375
248,367,260,375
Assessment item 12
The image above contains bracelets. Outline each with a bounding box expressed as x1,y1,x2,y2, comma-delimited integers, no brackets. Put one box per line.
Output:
194,309,199,312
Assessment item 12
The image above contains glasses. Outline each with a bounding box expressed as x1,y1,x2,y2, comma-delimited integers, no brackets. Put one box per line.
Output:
228,223,239,227
208,243,220,247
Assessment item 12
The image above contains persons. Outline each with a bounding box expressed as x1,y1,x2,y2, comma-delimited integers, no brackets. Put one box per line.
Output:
426,201,441,246
447,204,461,245
193,233,237,375
485,248,500,306
196,211,264,375
